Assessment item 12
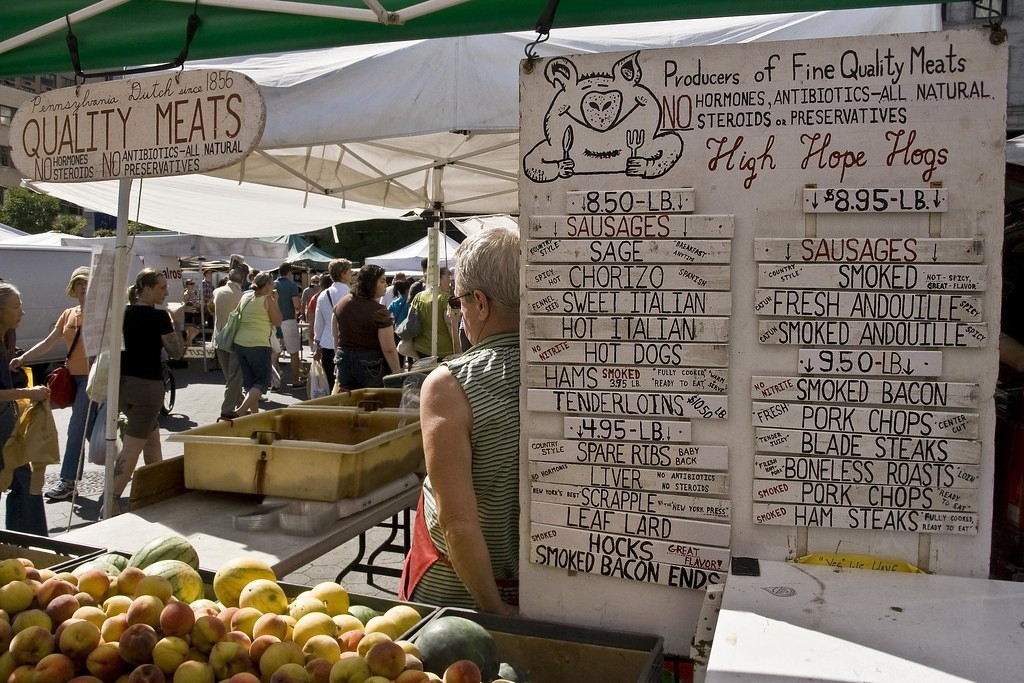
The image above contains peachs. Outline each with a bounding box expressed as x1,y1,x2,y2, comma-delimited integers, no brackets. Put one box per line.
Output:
0,557,515,683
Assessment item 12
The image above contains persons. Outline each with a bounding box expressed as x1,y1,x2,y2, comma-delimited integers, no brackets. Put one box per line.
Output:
0,248,466,520
401,227,520,619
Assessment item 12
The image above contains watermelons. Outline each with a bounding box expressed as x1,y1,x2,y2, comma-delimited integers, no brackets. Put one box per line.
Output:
71,534,203,608
346,605,380,625
413,616,501,683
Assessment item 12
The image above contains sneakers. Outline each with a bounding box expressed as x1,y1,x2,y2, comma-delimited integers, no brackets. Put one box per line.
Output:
45,479,79,499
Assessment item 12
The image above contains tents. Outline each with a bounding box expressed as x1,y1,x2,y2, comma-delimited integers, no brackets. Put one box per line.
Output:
0,0,971,80
30,4,941,519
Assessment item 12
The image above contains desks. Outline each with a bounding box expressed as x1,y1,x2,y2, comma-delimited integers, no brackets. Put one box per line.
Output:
46,483,423,586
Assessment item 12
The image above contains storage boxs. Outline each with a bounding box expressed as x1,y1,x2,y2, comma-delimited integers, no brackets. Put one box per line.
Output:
0,386,668,683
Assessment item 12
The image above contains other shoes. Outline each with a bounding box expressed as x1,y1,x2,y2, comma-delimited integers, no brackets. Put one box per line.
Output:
232,411,240,418
293,381,305,388
221,413,236,418
98,514,103,521
268,384,280,393
260,396,268,401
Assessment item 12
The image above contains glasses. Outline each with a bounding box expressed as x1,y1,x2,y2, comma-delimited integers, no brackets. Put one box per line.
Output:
190,283,195,285
446,292,471,309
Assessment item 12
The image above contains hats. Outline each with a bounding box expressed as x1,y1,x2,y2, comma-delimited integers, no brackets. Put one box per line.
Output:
311,275,319,287
65,266,90,297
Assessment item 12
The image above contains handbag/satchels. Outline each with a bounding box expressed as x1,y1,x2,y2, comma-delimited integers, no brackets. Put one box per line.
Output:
393,291,425,340
215,293,255,354
47,366,76,409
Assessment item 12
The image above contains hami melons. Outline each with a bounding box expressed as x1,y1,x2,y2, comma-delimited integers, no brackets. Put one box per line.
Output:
213,557,278,607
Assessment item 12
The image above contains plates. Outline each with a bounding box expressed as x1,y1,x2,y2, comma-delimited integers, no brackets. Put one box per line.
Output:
232,499,338,538
